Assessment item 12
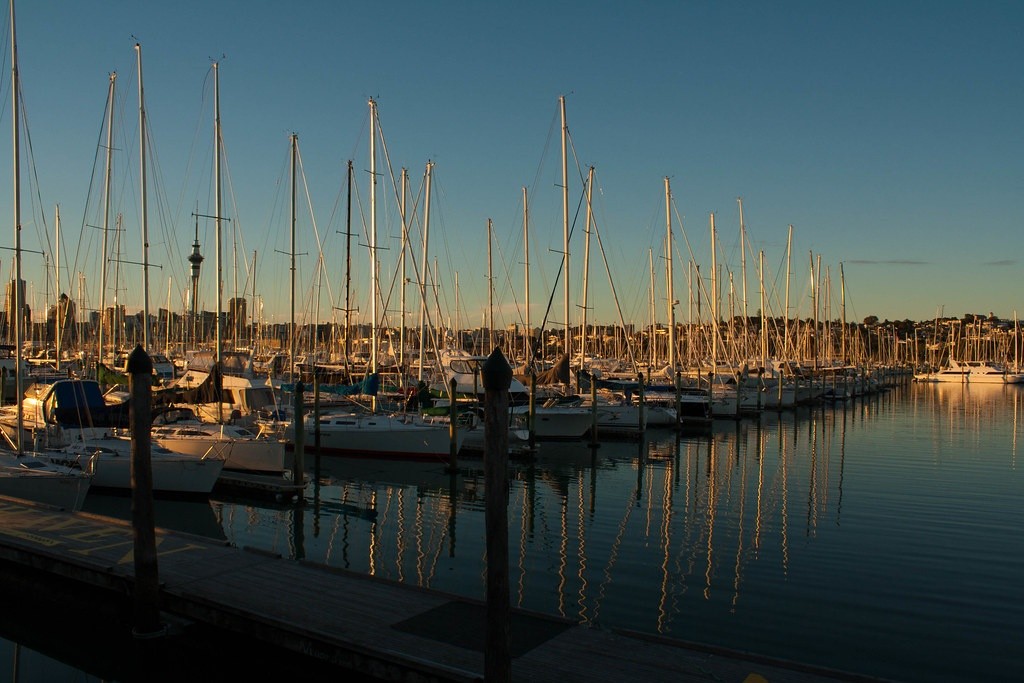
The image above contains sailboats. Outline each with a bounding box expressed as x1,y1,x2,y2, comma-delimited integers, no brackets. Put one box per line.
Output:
2,2,1024,522
